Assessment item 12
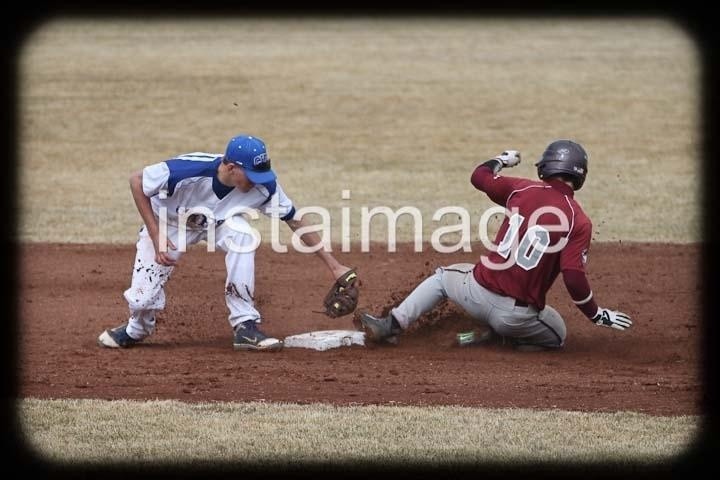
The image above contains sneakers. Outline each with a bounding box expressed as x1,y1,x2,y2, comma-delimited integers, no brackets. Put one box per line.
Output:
354,310,393,341
99,325,137,348
233,319,283,352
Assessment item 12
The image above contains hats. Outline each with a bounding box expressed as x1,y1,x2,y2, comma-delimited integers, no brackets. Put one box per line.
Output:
226,135,276,185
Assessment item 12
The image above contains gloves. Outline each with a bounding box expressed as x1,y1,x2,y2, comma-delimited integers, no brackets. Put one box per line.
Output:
497,150,521,168
591,307,632,331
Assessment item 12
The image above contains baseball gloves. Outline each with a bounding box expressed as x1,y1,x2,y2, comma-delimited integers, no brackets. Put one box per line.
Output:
322,268,359,319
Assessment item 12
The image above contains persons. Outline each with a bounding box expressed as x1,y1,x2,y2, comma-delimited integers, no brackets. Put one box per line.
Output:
355,139,635,348
97,136,362,355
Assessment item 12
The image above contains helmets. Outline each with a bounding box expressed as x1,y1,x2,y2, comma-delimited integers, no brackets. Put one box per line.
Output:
537,140,588,189
